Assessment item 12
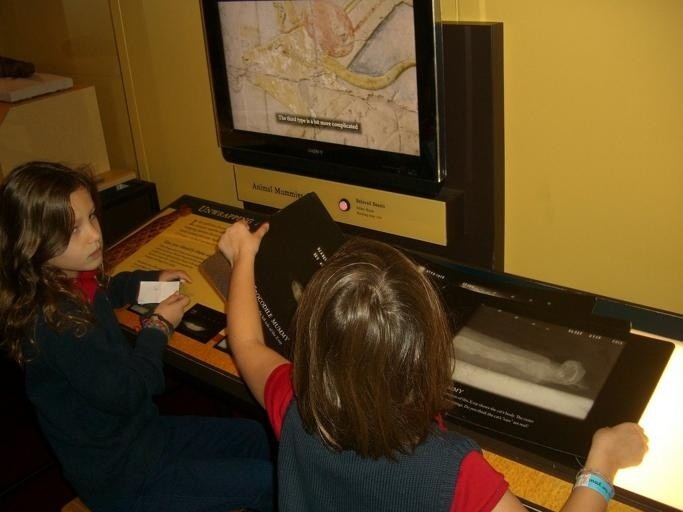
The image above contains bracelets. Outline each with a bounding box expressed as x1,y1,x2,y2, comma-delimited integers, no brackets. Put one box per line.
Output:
570,466,621,503
132,313,176,339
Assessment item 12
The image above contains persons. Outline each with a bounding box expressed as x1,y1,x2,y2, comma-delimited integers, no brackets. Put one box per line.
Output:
1,161,276,511
215,213,649,512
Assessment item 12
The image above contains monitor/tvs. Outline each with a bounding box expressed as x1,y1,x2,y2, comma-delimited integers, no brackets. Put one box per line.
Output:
199,0,447,183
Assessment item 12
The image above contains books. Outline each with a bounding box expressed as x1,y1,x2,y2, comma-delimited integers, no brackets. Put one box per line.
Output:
197,191,676,483
